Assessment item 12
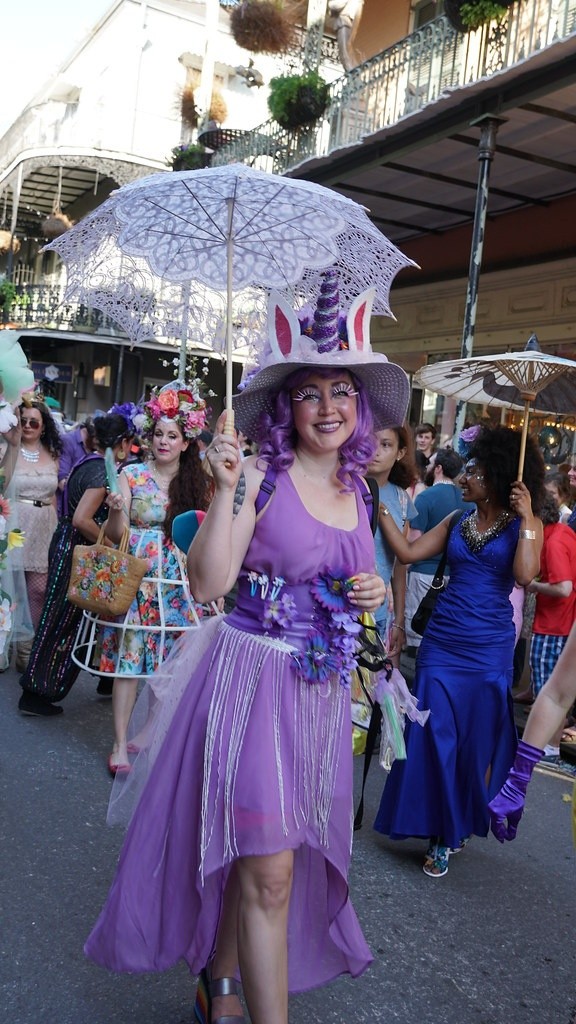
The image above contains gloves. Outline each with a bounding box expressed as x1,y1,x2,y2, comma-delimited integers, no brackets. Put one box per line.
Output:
486,736,546,843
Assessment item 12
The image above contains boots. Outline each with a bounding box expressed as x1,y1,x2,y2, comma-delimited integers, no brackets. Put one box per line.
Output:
14,628,35,673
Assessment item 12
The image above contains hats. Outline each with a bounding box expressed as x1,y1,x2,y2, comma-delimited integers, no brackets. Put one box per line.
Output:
222,270,411,440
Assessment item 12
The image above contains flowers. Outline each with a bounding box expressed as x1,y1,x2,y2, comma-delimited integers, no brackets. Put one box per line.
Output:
142,345,219,440
258,591,299,632
291,563,362,690
0,492,26,572
106,400,147,436
162,140,206,170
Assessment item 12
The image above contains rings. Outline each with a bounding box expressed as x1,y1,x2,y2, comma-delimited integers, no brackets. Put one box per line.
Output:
215,446,219,453
117,503,120,507
512,495,514,500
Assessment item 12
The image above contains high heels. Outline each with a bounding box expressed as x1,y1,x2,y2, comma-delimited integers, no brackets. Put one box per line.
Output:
193,957,247,1024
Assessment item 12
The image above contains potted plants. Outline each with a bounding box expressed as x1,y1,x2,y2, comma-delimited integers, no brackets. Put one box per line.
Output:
39,212,75,244
0,230,22,257
169,78,228,131
0,275,17,313
266,66,333,137
442,0,518,34
228,0,300,58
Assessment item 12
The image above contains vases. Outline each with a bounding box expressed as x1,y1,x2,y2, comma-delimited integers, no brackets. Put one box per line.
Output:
172,153,212,171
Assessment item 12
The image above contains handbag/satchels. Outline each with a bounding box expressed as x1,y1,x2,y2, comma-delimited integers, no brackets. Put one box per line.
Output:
412,508,465,636
66,518,147,617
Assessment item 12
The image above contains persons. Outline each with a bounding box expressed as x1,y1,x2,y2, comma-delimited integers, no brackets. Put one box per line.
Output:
82,269,411,1024
328,0,365,95
349,422,576,877
0,378,257,778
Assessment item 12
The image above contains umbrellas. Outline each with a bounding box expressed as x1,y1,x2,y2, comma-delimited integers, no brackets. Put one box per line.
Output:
37,163,421,434
415,334,576,489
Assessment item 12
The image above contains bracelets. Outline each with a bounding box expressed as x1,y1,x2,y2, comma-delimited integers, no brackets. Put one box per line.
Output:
519,530,535,539
392,623,405,631
383,508,390,516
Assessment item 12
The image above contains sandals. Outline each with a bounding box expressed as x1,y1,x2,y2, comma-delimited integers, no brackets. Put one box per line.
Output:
423,832,451,879
448,833,476,855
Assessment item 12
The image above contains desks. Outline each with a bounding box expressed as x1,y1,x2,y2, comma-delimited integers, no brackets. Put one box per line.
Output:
196,129,284,167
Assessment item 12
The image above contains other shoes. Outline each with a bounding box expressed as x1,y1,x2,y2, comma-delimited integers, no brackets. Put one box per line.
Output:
402,645,416,659
127,743,151,753
98,678,114,698
17,691,64,716
109,753,132,774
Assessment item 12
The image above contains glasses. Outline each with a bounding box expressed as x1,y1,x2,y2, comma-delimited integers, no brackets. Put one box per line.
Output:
20,418,43,429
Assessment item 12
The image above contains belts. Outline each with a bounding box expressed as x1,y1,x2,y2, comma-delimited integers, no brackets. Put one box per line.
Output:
13,498,51,508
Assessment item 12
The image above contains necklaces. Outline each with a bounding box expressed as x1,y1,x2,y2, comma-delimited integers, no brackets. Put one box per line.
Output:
433,480,455,486
460,509,510,552
21,442,41,463
153,460,179,489
